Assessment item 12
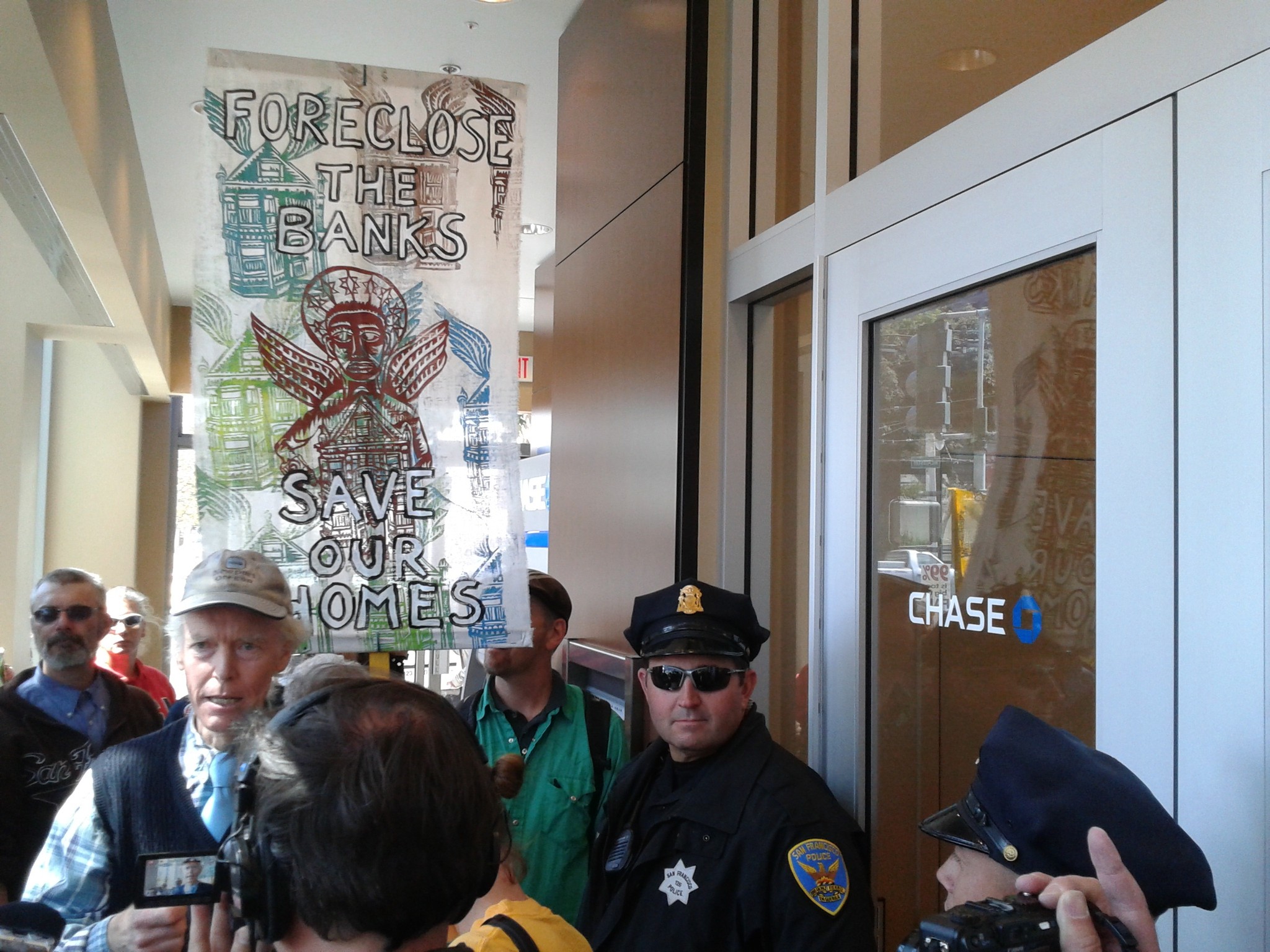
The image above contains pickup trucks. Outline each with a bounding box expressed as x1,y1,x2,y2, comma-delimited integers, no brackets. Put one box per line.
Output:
877,549,950,599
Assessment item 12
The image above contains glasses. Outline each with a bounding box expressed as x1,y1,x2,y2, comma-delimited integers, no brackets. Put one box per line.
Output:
109,615,145,628
33,603,104,624
647,665,747,692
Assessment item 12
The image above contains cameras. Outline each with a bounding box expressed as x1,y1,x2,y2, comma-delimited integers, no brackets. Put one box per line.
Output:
893,892,1098,952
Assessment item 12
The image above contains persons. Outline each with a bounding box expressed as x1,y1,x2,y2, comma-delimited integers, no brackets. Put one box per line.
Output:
1015,827,1160,952
20,552,308,952
577,581,879,952
454,570,631,930
188,677,593,952
918,703,1217,920
94,586,176,719
284,655,368,703
156,857,212,895
1,568,165,952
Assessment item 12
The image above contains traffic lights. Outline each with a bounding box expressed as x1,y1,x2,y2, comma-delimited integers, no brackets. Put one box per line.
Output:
905,321,951,435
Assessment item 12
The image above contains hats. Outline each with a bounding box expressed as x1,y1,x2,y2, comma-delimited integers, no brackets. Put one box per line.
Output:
169,548,293,618
624,578,771,659
918,705,1218,925
527,567,572,615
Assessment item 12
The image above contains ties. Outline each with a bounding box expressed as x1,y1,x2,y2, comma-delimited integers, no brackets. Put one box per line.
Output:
197,746,241,846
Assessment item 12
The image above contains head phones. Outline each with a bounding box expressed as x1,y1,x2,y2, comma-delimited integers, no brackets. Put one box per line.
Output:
216,679,513,947
